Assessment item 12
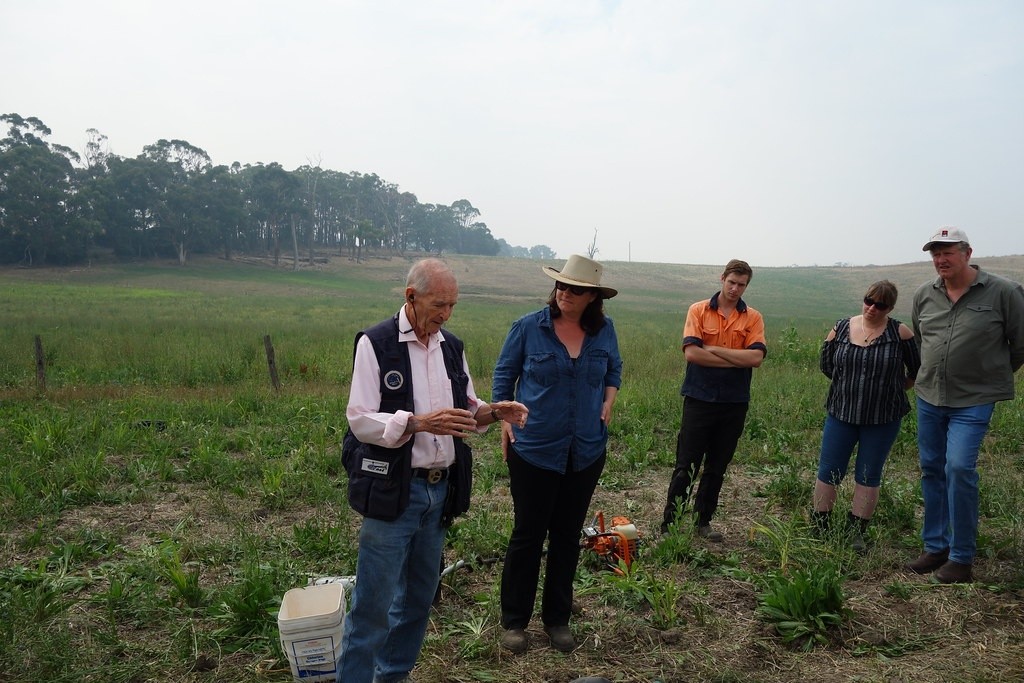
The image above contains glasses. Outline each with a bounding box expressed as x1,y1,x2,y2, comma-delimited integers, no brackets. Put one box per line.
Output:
863,296,891,311
555,280,593,296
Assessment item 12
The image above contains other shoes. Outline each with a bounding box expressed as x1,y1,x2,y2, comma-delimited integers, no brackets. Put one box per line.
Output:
659,520,675,536
693,524,722,542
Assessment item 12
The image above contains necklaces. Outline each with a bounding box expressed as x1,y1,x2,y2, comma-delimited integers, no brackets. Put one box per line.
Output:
862,316,873,342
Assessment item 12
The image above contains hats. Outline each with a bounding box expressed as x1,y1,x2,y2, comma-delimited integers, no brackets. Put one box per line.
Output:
541,255,618,300
922,224,969,251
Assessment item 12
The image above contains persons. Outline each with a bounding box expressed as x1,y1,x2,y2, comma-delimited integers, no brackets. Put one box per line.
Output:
660,259,766,538
813,280,918,550
336,257,529,683
909,229,1024,582
491,256,622,647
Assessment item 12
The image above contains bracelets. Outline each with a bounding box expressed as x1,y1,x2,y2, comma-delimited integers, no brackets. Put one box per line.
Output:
491,409,499,421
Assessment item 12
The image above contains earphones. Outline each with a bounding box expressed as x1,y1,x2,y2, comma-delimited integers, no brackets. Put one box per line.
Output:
409,294,415,303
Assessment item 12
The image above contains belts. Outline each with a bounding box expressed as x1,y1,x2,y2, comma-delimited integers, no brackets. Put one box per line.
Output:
411,466,451,485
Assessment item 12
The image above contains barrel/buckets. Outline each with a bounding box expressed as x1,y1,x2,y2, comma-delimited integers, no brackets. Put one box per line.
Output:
277,582,345,683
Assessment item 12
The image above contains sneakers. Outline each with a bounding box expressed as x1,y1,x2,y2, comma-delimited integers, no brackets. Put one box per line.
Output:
501,625,527,652
811,509,832,541
544,624,575,651
845,511,867,553
905,546,950,574
936,560,972,583
